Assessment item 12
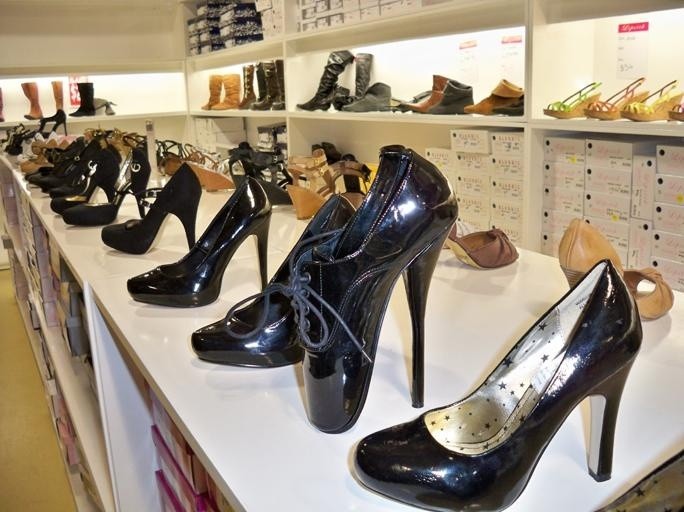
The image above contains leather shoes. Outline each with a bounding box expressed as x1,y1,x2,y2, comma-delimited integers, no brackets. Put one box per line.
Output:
425,79,475,114
398,75,452,113
491,93,523,116
462,79,523,114
191,192,359,369
340,82,391,114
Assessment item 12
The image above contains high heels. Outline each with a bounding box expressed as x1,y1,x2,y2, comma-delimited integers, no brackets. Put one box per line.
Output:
667,101,684,124
292,144,458,434
101,162,202,255
127,175,273,308
558,217,675,321
0,109,236,226
619,81,684,122
541,80,602,119
356,260,643,511
228,154,294,206
584,75,648,120
284,149,336,220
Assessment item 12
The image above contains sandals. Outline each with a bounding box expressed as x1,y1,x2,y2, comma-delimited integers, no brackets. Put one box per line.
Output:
446,217,519,270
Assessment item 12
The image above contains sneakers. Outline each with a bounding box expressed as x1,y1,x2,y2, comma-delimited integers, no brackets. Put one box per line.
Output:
311,142,341,160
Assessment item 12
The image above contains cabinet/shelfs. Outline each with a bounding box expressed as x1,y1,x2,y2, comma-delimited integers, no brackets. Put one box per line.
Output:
1,1,684,512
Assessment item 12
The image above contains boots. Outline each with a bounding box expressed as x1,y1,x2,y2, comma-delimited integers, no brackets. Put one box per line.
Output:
271,60,285,110
256,63,266,102
210,74,240,110
237,65,257,109
200,74,221,111
67,83,96,117
92,96,115,116
295,48,354,112
20,82,43,120
50,81,64,116
249,62,279,111
349,52,373,99
